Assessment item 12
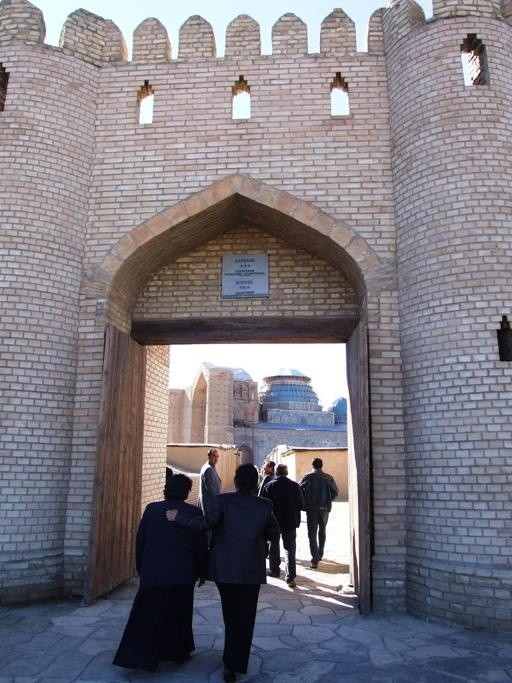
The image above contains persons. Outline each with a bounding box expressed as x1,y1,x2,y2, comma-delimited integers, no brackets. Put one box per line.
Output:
196,449,222,548
110,473,208,674
260,464,304,589
165,462,281,682
258,461,276,556
299,458,340,568
